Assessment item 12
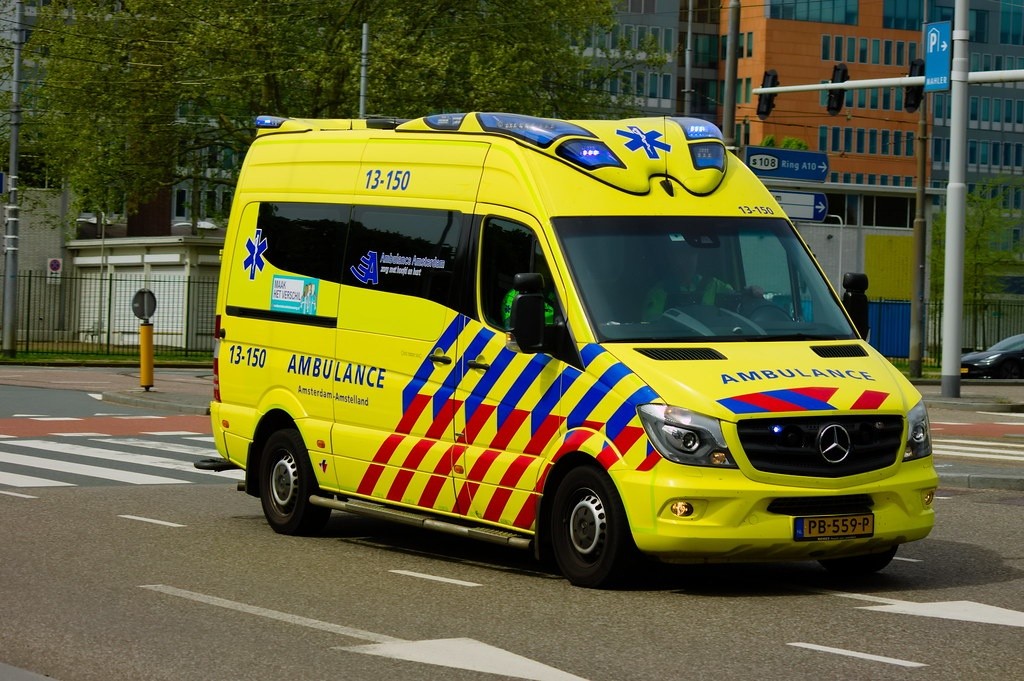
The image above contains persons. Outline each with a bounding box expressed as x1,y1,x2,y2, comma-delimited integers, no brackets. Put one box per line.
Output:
500,267,558,328
639,242,764,324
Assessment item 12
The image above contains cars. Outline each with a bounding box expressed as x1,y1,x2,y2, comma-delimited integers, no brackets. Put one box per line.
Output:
958,332,1024,381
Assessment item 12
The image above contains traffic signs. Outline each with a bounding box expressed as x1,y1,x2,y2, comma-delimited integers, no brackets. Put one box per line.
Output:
745,146,830,185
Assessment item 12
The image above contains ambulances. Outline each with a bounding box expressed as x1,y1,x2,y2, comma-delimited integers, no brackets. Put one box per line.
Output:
190,105,941,594
